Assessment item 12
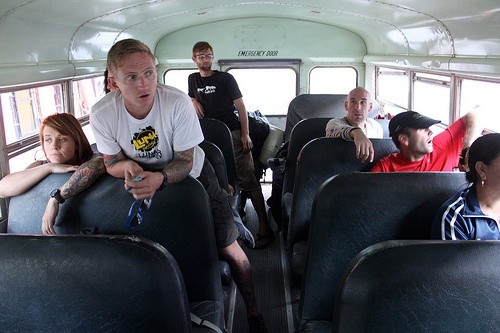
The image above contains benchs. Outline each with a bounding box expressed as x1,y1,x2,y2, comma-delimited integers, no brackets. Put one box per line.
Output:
280,94,500,333
0,115,240,333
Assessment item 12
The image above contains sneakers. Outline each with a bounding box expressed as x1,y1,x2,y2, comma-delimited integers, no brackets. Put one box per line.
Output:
231,208,255,249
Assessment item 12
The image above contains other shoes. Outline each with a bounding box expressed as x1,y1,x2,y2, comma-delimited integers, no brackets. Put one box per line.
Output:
253,231,275,248
248,312,268,333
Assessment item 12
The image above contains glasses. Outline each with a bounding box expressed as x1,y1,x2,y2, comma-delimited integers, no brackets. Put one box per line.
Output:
126,176,152,229
196,54,214,59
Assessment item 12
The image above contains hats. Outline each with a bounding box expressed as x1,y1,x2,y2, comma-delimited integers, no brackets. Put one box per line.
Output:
389,110,441,137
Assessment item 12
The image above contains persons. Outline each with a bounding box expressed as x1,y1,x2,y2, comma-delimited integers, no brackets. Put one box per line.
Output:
187,41,275,249
104,64,257,249
0,113,105,236
324,86,384,163
90,39,263,333
370,105,479,173
427,133,500,241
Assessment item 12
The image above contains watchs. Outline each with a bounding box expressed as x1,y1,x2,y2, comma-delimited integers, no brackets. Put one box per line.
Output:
158,170,168,191
50,188,65,205
